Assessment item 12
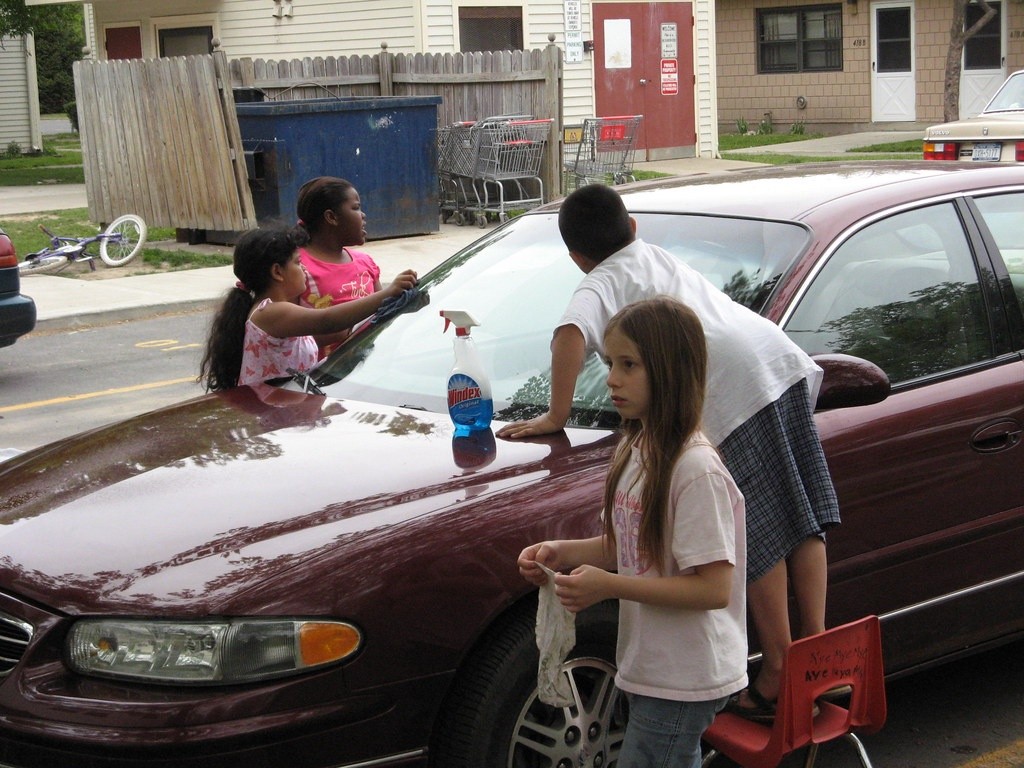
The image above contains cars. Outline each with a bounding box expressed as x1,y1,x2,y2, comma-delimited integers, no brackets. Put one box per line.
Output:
0,159,1024,768
922,70,1024,161
0,228,37,348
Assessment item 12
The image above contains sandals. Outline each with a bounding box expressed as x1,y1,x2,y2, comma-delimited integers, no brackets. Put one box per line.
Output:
726,687,819,727
816,684,854,702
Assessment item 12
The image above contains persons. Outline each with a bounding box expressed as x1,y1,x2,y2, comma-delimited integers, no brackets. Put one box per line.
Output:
289,176,379,362
197,222,417,393
495,184,853,719
515,299,750,767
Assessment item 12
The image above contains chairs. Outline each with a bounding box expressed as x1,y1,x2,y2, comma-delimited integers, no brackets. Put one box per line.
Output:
848,267,962,385
700,614,887,768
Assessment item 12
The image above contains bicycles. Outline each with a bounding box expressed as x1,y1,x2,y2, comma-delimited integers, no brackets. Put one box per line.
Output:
17,214,147,278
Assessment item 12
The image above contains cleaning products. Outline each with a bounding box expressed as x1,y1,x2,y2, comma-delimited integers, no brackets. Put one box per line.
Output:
446,430,498,481
438,309,494,429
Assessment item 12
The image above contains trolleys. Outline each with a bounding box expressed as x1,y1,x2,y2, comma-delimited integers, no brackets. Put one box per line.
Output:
438,114,555,230
561,114,643,191
